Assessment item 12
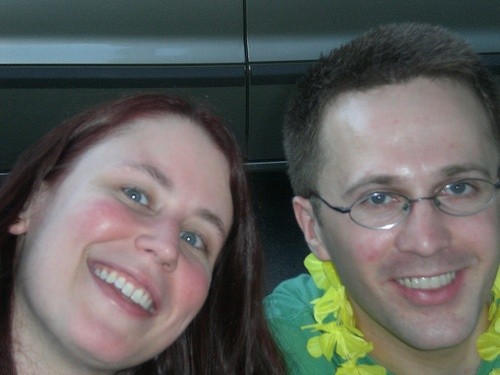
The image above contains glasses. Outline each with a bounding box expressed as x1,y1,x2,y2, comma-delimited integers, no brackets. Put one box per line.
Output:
304,170,500,231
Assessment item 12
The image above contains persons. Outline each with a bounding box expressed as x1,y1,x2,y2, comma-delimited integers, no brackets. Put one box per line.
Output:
1,89,295,374
260,19,499,375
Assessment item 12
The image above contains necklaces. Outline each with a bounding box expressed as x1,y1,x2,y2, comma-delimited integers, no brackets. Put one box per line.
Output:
302,250,499,375
